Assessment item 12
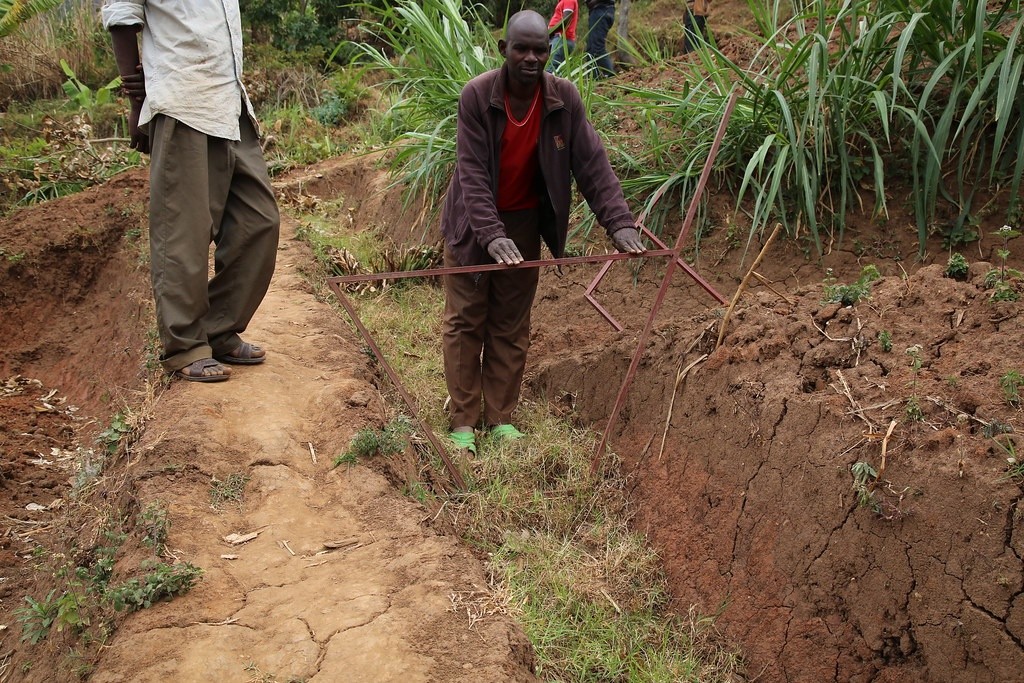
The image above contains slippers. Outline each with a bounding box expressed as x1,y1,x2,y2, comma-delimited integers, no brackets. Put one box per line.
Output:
174,358,230,381
450,432,477,461
490,425,526,440
215,340,266,363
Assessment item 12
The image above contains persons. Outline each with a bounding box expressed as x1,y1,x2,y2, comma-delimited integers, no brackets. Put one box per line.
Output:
683,0,713,54
440,10,646,462
547,0,578,74
586,0,615,81
101,0,280,383
